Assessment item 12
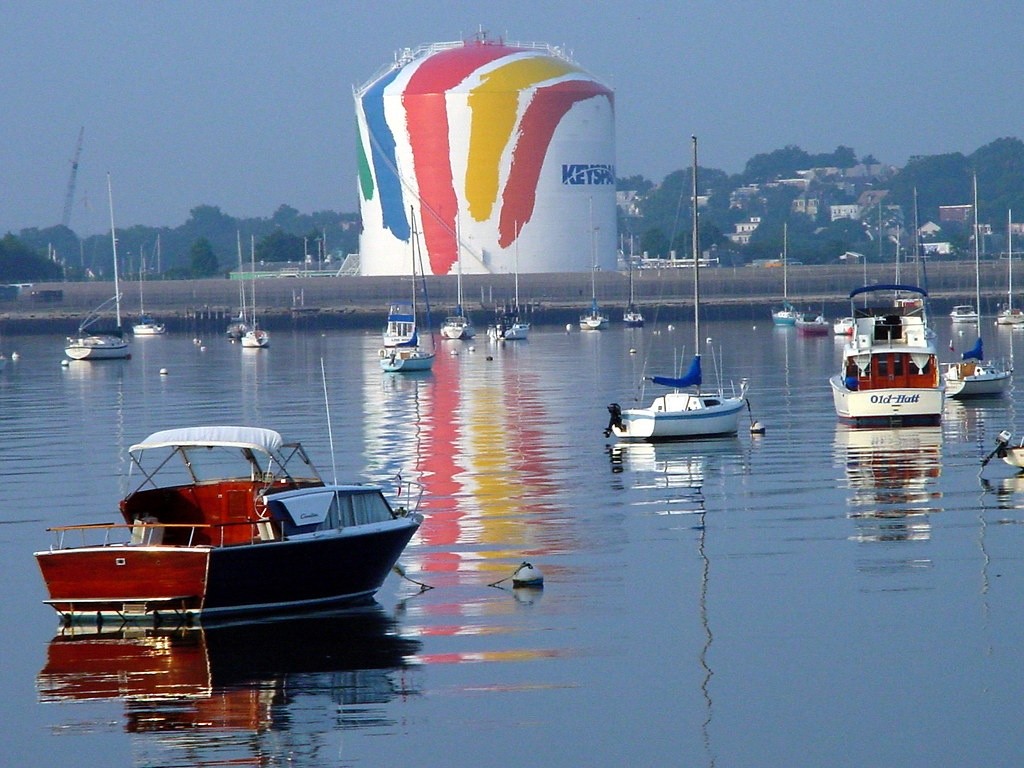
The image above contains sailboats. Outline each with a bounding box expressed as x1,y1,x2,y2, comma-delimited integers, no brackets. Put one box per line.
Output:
64,172,132,361
997,208,1024,325
375,206,437,371
623,234,645,328
940,173,1013,397
132,246,165,334
439,208,476,341
579,197,611,330
605,135,751,439
226,230,269,347
487,220,532,340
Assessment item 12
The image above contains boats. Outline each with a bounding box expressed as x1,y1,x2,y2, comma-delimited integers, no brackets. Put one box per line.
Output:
949,304,978,323
770,187,945,422
33,357,425,624
980,427,1024,468
